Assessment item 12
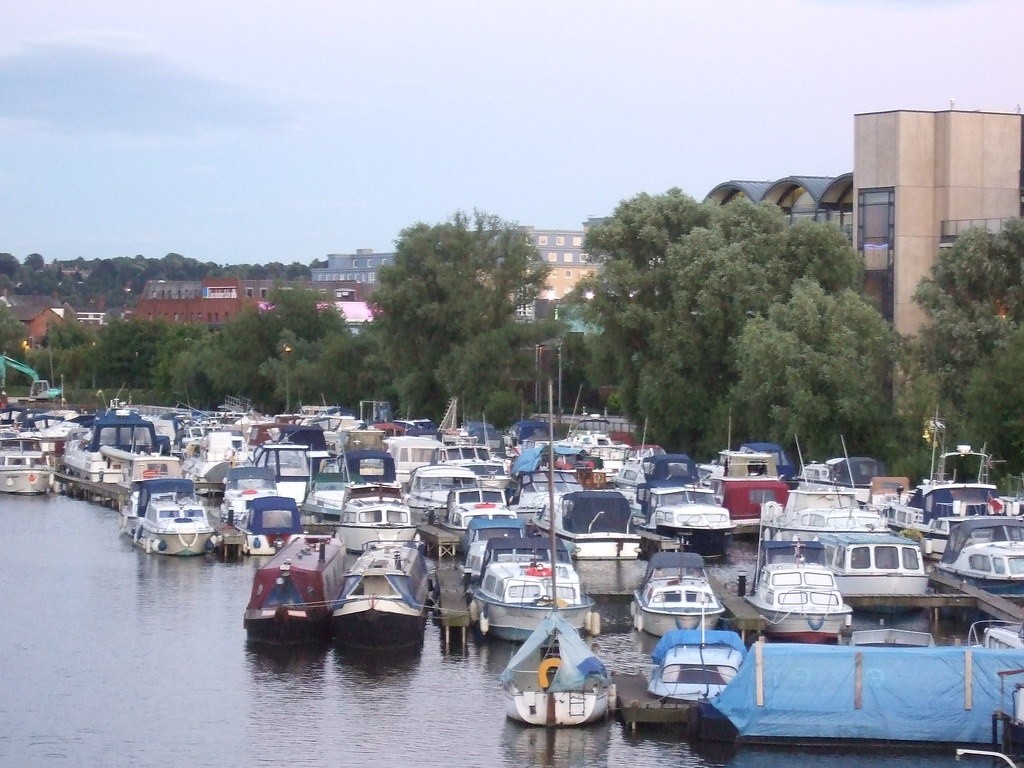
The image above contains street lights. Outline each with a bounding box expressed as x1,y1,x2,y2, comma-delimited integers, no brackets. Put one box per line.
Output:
283,344,292,414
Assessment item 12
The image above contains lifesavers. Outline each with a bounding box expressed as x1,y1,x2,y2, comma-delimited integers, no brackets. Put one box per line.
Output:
242,489,257,494
525,567,552,576
474,504,495,508
537,658,561,687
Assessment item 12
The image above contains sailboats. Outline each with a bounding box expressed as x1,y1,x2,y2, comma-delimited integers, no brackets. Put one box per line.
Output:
498,380,618,728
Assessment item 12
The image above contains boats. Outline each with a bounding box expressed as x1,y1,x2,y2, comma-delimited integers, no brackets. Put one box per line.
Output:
760,490,931,615
467,536,596,644
648,588,746,703
117,477,214,557
243,535,347,647
1,398,1024,559
633,551,726,638
743,492,854,645
932,518,1024,624
329,539,433,647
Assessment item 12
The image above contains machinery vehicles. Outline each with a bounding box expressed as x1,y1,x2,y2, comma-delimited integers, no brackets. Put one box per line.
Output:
0,354,62,402
